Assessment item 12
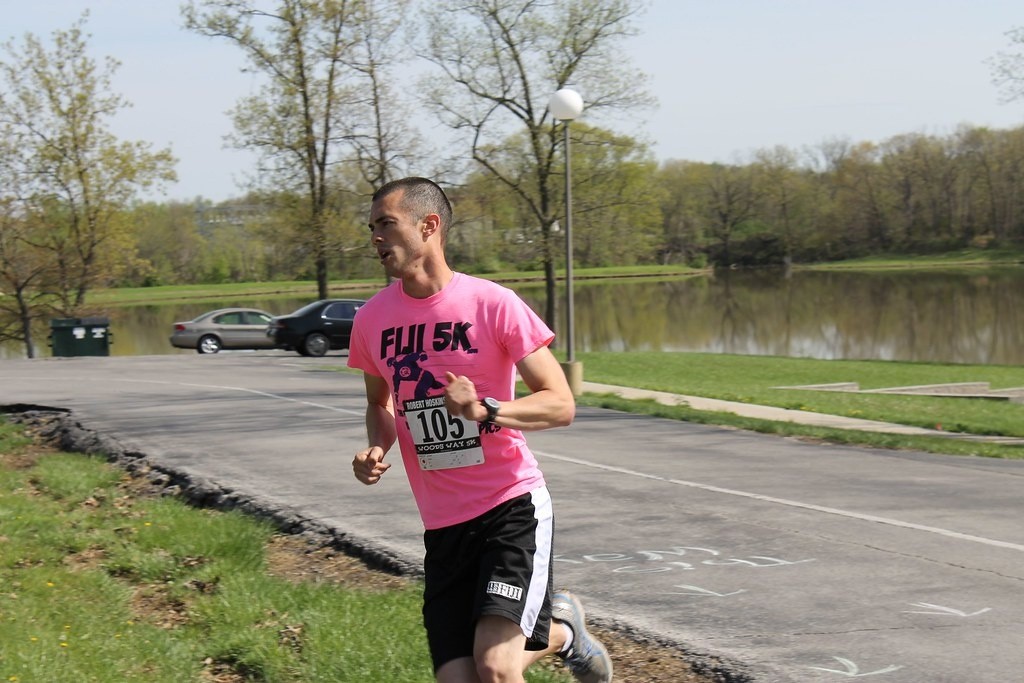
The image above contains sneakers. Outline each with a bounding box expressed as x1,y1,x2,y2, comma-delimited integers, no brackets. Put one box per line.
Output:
551,590,613,683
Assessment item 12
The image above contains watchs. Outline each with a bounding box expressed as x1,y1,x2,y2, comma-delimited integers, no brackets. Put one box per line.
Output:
480,396,500,425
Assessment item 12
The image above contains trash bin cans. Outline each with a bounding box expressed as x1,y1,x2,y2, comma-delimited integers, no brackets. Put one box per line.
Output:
47,315,114,356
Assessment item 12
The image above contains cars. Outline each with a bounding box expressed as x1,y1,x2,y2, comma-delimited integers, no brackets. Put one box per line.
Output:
168,307,284,354
264,299,368,357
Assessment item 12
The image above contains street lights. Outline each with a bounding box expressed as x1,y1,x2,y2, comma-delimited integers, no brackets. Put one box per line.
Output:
547,87,585,397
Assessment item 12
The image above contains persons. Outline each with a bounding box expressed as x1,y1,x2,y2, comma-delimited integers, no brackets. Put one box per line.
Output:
347,176,612,682
